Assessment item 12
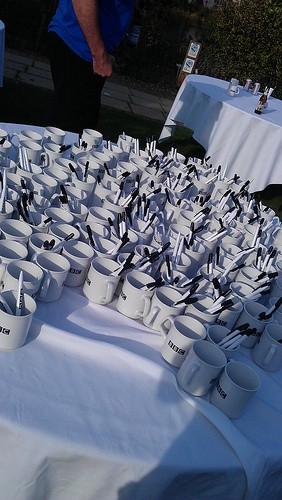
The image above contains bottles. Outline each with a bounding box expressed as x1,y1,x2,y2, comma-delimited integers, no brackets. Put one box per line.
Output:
254,87,269,114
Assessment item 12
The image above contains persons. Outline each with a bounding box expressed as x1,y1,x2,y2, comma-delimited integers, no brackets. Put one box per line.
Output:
47,0,136,137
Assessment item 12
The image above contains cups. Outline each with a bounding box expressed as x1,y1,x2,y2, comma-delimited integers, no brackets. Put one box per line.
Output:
226,78,240,97
0,126,281,418
265,86,274,99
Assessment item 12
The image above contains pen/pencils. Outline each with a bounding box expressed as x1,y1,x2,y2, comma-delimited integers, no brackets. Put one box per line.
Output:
79,131,87,151
86,225,100,252
83,161,89,181
37,217,52,227
139,274,282,350
44,239,55,250
55,145,71,153
17,180,35,226
108,217,119,238
69,163,82,181
0,137,8,213
208,253,213,274
58,185,72,212
41,240,49,250
139,213,156,233
16,271,23,316
0,295,14,315
104,132,278,283
51,233,74,250
19,148,31,173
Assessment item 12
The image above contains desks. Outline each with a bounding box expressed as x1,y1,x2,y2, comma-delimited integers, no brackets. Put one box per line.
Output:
159,74,282,194
0,123,282,500
